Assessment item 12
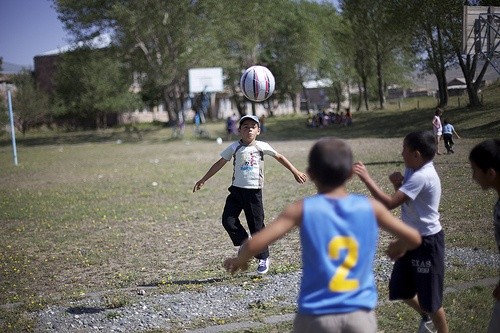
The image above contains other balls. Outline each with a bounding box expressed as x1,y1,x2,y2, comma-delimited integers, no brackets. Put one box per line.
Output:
216,137,223,146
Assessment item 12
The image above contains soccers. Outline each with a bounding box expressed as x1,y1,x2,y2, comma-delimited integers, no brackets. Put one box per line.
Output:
240,65,276,103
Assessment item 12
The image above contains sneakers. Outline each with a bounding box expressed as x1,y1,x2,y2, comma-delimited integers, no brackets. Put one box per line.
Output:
257,257,268,274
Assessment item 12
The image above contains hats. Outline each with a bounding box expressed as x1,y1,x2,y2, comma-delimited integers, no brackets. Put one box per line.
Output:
239,116,260,129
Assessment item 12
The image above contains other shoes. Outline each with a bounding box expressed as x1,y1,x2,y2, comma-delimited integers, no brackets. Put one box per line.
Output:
419,319,438,333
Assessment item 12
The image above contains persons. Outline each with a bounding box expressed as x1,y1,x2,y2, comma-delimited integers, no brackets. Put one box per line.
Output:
227,113,238,140
192,113,201,126
260,115,265,131
442,118,458,155
193,115,306,275
432,108,443,155
351,131,450,333
312,108,352,129
177,112,185,134
468,139,500,332
224,138,423,333
